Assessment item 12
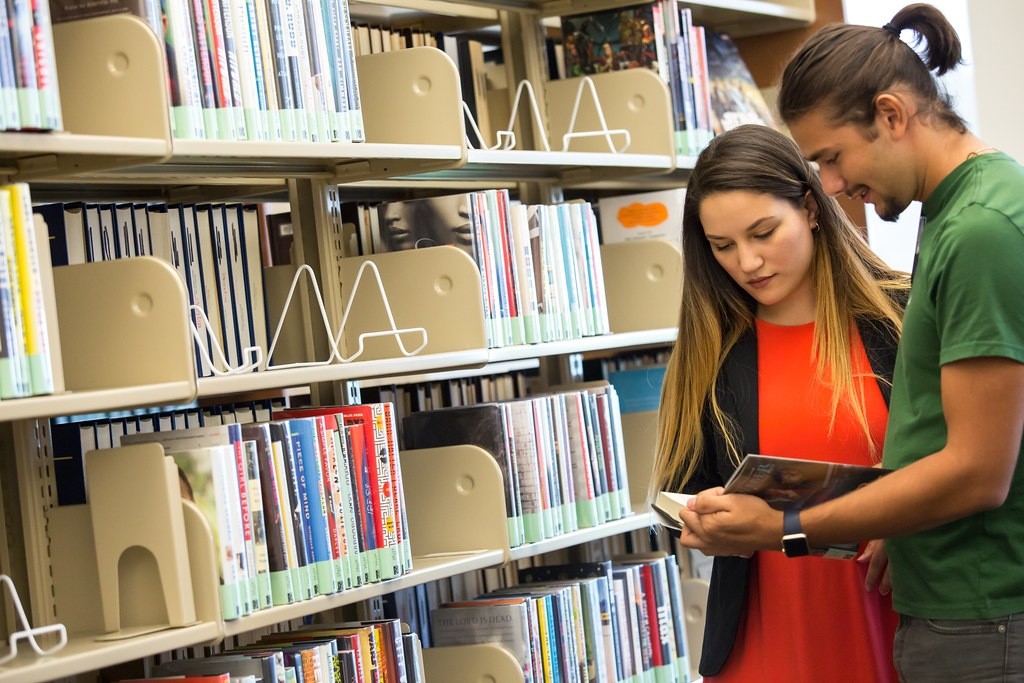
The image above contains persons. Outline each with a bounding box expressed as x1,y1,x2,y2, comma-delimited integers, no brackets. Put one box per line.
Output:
421,193,471,257
378,200,418,255
650,124,910,683
681,3,1024,683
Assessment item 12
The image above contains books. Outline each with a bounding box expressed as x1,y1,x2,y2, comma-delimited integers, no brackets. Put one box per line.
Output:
652,453,895,559
0,0,776,683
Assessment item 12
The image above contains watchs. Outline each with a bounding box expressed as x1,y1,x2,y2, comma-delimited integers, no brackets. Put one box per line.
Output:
782,508,809,557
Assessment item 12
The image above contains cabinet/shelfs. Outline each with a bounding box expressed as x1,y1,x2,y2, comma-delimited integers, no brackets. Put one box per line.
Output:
1,0,867,683
